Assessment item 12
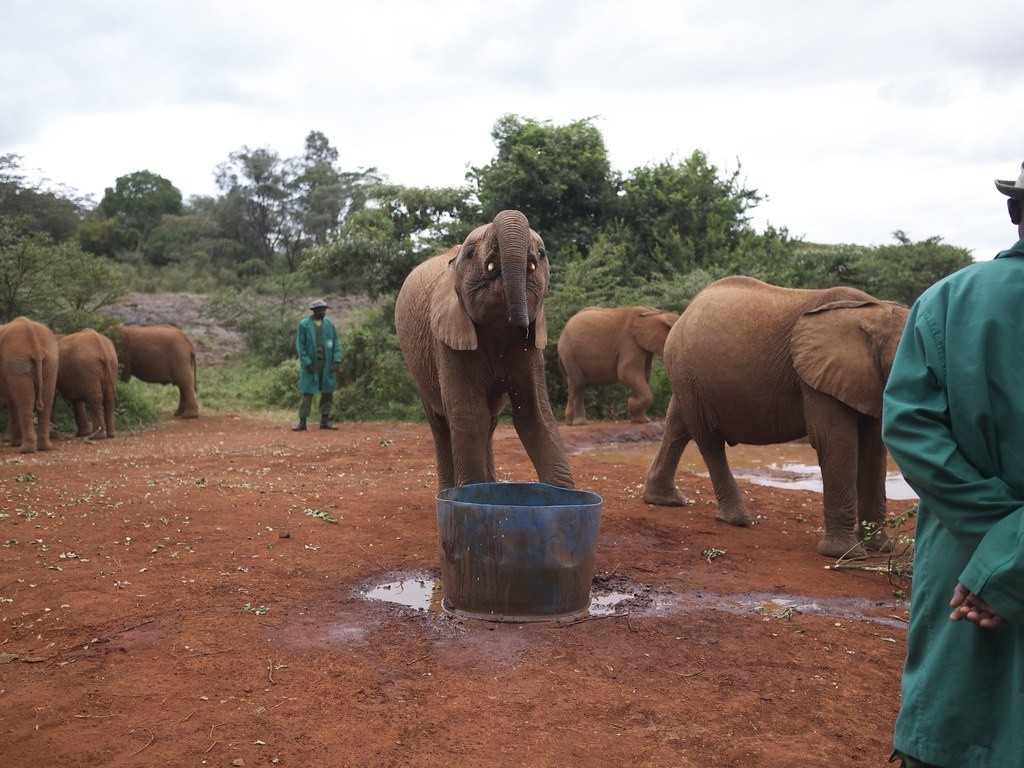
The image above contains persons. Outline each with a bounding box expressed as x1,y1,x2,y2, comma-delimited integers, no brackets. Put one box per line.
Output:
291,300,341,431
879,162,1023,767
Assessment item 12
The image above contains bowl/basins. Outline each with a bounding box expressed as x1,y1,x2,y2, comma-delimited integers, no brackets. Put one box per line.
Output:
433,482,604,625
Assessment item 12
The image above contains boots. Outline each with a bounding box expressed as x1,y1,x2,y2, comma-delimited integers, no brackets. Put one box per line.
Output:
292,418,307,431
320,416,337,430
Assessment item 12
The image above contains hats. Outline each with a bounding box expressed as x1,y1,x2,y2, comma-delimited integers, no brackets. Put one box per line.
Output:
995,161,1024,200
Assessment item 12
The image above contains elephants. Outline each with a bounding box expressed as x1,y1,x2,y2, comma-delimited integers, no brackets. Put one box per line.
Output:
0,316,199,454
557,305,680,425
644,275,911,558
394,209,574,492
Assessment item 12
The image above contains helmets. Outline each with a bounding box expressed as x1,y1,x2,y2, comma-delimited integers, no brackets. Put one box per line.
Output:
309,300,330,310
